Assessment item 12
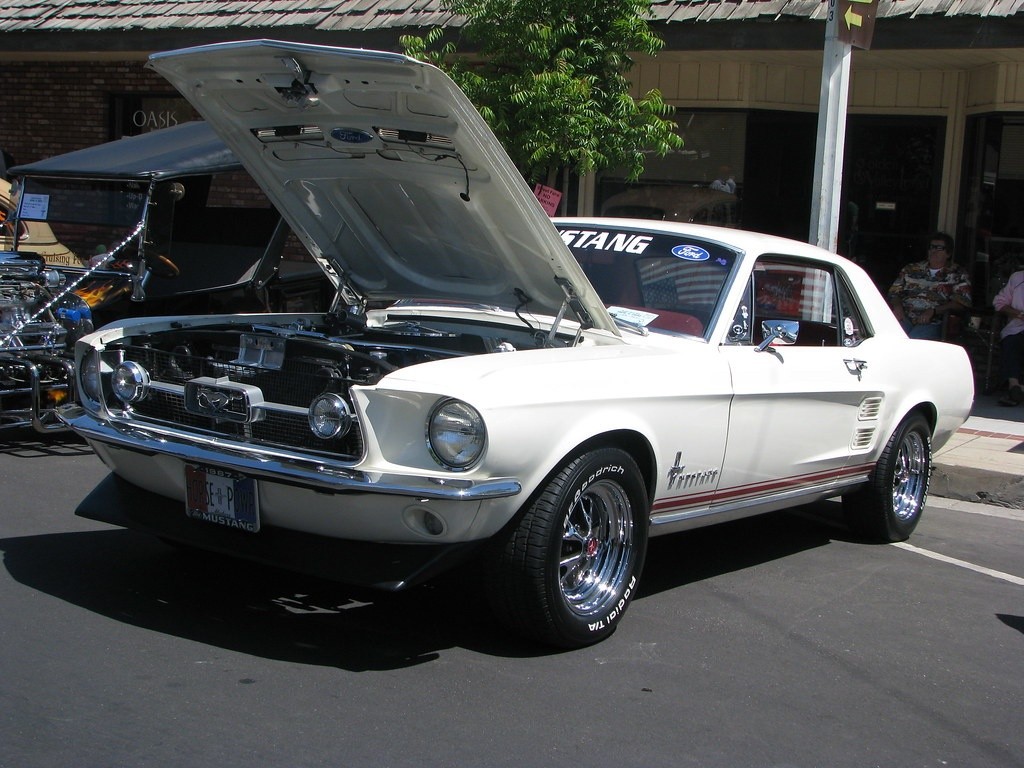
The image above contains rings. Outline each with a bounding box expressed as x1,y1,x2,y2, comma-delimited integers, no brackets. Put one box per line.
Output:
923,319,925,322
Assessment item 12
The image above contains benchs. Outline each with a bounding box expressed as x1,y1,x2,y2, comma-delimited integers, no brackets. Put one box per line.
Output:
145,239,268,295
658,308,839,348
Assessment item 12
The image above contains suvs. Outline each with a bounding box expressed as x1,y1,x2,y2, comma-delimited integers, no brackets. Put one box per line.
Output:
3,122,331,434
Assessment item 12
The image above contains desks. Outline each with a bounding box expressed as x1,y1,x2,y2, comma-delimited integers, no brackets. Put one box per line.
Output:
940,308,1006,394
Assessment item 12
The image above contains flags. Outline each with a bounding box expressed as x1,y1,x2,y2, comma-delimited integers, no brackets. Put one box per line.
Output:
638,258,730,310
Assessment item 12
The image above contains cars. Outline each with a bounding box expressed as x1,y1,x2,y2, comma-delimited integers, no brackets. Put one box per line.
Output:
0,175,90,269
54,39,974,646
597,185,745,227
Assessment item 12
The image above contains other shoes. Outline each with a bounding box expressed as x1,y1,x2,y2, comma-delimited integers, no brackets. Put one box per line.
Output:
998,386,1024,406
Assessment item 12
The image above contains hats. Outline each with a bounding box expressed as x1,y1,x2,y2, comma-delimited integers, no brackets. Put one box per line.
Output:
95,245,106,255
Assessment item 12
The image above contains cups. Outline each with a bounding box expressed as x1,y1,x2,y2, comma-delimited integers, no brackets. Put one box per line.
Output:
971,316,980,329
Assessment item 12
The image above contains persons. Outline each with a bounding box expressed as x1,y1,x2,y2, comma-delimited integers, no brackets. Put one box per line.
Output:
709,166,736,194
887,233,972,340
993,270,1024,407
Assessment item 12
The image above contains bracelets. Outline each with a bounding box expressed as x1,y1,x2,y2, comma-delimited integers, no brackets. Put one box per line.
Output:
933,308,936,317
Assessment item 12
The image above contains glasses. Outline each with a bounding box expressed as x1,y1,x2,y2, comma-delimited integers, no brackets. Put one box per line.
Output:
929,244,946,252
